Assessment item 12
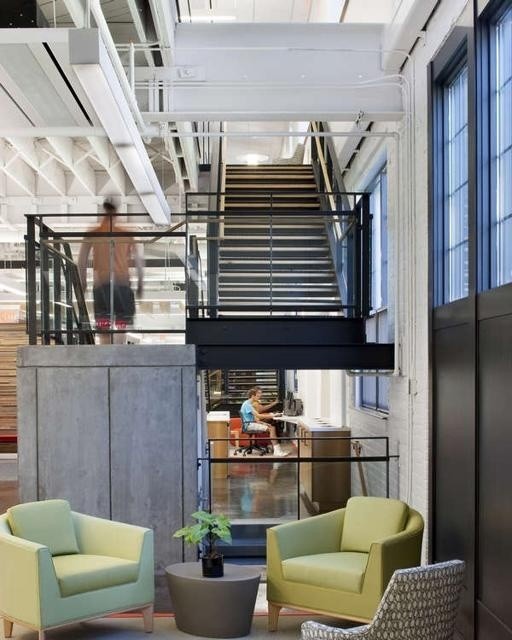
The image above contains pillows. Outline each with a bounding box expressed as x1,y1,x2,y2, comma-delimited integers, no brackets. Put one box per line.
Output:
340,497,411,554
6,499,79,557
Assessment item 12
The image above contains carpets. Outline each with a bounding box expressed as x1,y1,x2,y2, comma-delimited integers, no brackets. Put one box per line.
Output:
104,582,322,617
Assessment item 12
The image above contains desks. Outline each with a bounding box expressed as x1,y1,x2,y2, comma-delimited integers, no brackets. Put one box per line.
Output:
270,413,297,424
164,563,261,638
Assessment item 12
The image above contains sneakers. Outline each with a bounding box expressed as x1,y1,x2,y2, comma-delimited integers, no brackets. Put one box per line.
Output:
274,450,291,457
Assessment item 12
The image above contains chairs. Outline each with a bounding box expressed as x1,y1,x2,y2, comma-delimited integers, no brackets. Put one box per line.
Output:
266,496,424,632
230,411,267,456
0,499,155,640
301,559,466,640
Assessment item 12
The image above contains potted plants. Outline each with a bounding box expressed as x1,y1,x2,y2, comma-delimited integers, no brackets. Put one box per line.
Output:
173,511,232,578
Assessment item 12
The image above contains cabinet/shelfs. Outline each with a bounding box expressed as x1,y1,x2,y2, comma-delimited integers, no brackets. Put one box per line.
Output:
207,411,230,478
295,416,351,517
17,344,198,611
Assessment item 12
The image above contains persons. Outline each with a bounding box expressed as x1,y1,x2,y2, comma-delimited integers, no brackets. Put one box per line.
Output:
240,389,289,456
77,195,144,345
253,386,280,421
